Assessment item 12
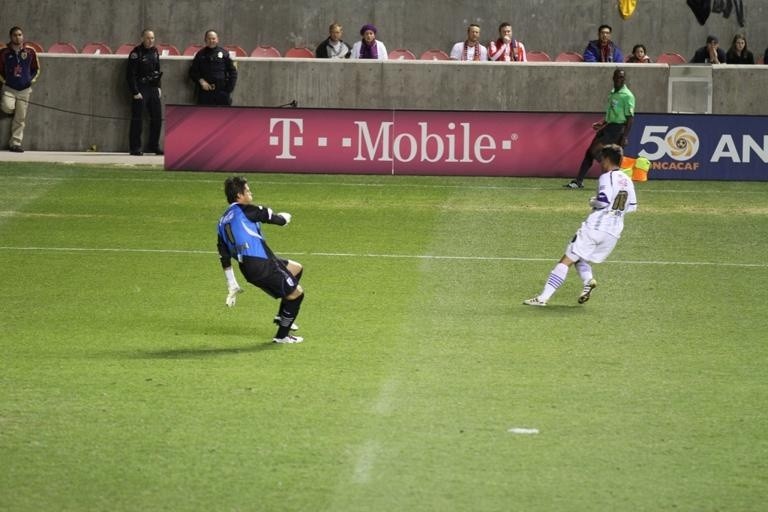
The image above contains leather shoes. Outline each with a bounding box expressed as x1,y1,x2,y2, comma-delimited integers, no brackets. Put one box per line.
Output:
129,145,164,155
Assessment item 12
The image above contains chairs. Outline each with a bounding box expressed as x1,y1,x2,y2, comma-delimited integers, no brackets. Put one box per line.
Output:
23,41,139,54
390,48,450,62
158,43,314,60
623,52,684,63
528,50,585,63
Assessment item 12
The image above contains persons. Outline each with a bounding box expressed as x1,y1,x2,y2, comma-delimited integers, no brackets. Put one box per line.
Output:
315,23,351,58
725,33,754,64
694,35,726,64
563,68,636,190
189,29,238,107
584,24,624,62
449,24,488,61
487,22,527,61
764,48,768,65
126,29,164,156
626,44,651,63
0,26,40,152
218,176,304,344
523,144,638,310
349,24,388,60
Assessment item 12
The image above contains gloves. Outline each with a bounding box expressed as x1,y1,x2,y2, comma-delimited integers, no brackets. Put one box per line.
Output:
277,212,292,227
224,285,245,308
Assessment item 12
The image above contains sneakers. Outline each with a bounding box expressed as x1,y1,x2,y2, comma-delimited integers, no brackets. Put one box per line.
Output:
8,144,25,152
522,297,547,307
562,179,585,189
577,278,597,304
274,315,299,331
272,334,304,344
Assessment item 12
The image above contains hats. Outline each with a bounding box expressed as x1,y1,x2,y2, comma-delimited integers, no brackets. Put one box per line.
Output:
360,24,377,35
706,35,719,43
